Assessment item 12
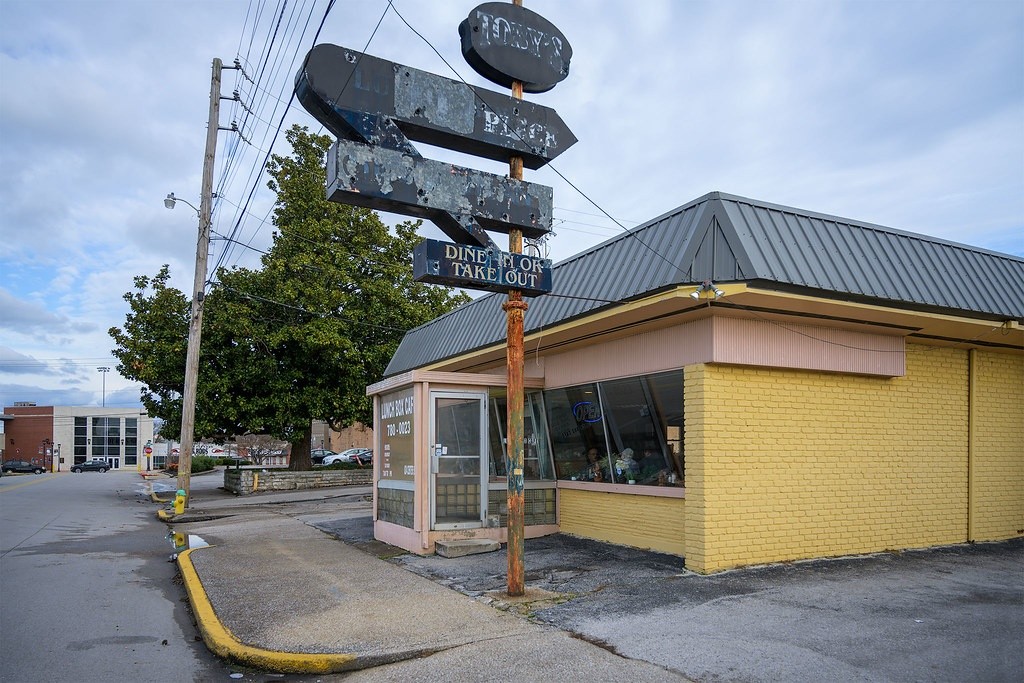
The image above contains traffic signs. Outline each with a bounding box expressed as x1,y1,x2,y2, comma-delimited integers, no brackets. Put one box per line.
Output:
292,45,581,171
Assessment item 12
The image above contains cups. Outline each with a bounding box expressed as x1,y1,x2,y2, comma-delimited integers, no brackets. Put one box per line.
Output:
629,480,635,484
572,477,576,480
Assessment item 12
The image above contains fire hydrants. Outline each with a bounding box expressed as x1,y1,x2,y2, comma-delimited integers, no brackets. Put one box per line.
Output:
170,490,187,515
171,532,186,551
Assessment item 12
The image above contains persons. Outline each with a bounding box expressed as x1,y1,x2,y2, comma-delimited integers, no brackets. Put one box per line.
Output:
589,449,599,465
621,448,640,475
638,444,666,475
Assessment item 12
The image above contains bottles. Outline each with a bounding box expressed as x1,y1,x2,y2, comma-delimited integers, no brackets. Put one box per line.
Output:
671,471,676,483
668,473,672,482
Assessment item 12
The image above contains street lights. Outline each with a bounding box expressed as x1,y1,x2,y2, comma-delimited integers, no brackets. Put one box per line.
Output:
163,192,212,511
58,444,61,472
96,367,110,407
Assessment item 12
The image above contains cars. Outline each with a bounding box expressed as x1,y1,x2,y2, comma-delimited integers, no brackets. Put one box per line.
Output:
171,447,224,455
310,449,339,467
322,448,369,465
348,450,374,467
2,460,47,474
70,461,110,475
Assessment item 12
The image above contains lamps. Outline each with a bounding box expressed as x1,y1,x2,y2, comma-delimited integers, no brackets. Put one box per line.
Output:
87,438,91,445
689,280,726,302
121,439,124,445
10,438,15,445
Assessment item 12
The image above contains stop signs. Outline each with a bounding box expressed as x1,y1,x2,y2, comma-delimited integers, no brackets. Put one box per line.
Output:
43,440,46,445
145,448,152,454
51,442,54,446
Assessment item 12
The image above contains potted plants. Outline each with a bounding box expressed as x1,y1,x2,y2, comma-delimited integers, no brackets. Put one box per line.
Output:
623,472,638,484
